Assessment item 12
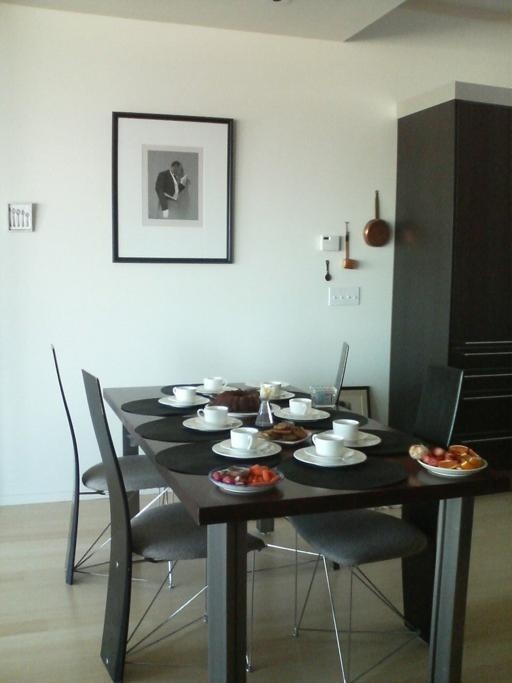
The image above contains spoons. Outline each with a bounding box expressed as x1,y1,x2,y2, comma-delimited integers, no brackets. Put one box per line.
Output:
325,259,332,281
12,207,31,228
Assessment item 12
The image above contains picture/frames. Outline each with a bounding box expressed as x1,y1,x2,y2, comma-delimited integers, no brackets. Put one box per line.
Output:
109,110,236,267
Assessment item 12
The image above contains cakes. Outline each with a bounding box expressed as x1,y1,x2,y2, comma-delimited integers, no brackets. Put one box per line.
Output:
209,391,261,412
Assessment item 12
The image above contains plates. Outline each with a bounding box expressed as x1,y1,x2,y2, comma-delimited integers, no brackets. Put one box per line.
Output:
277,407,330,421
325,430,382,449
221,440,268,451
168,396,203,403
182,416,243,433
417,456,489,477
196,383,240,393
203,402,280,416
212,439,283,460
157,394,209,408
293,445,369,468
262,428,311,444
210,487,285,503
304,447,354,459
258,389,295,400
417,470,459,485
209,470,285,492
245,381,289,388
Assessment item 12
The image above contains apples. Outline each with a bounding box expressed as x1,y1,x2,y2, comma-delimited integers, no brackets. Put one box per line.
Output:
422,447,446,466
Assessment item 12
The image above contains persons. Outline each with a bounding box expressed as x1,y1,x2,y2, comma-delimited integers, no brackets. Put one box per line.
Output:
173,163,191,217
156,161,180,219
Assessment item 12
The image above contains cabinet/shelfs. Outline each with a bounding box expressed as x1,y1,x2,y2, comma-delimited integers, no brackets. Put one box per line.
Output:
385,99,511,497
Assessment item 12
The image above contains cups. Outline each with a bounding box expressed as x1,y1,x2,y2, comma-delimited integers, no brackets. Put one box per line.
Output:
198,403,230,426
172,385,197,400
289,398,312,415
332,418,360,441
311,433,345,457
268,382,288,398
231,426,259,450
309,385,337,409
202,375,226,391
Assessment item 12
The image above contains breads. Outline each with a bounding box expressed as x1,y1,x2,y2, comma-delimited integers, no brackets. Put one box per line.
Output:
260,421,308,441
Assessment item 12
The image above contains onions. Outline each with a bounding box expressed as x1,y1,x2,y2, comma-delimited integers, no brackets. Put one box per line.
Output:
213,472,235,484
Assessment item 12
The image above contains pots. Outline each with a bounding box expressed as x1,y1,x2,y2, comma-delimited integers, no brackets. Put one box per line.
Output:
362,188,390,247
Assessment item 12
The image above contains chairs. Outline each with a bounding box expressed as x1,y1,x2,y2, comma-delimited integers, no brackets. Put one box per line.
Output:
80,371,265,682
49,342,174,588
262,340,351,572
284,359,474,683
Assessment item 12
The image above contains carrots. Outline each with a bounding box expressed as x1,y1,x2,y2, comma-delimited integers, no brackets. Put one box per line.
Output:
247,464,280,485
